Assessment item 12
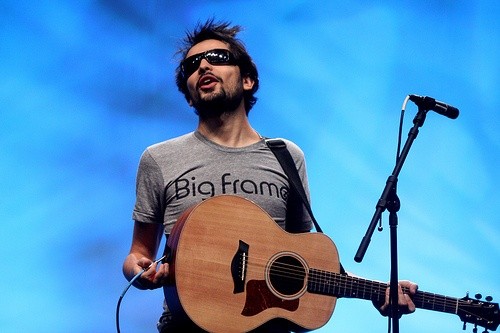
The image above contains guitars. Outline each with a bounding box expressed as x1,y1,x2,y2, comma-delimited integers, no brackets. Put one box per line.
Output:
164,194,500,333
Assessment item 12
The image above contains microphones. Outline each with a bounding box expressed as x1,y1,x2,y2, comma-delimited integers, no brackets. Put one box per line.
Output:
409,94,459,119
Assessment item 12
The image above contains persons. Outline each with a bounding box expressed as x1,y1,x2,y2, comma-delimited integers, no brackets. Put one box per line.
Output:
122,14,419,333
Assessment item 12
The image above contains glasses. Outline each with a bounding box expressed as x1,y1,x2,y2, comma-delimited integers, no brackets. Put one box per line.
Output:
180,47,239,78
